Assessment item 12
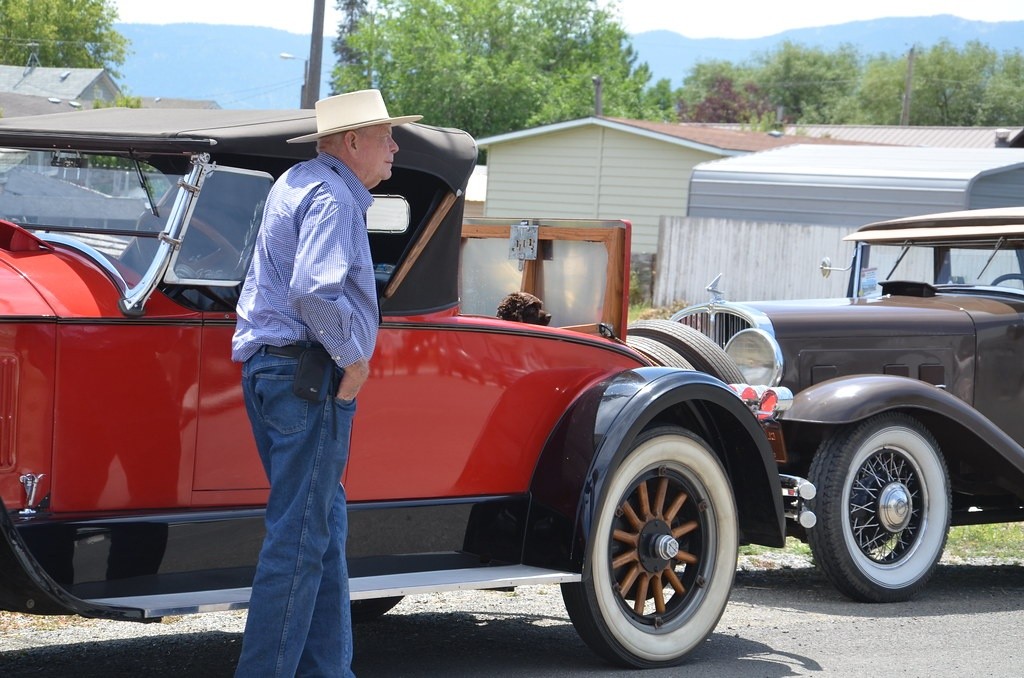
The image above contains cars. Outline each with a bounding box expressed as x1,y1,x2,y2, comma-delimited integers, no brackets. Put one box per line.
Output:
669,206,1024,604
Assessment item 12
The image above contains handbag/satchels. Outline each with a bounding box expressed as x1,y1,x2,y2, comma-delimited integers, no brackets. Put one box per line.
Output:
293,346,335,403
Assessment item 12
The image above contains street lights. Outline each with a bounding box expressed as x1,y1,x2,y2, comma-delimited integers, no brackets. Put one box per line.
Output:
279,53,310,109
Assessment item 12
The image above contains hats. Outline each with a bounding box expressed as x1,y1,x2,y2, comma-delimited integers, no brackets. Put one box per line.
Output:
286,89,424,143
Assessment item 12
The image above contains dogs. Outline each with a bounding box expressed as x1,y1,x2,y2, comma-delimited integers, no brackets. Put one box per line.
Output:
494,291,553,328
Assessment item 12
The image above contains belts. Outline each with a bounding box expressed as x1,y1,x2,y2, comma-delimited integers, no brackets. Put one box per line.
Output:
258,345,306,358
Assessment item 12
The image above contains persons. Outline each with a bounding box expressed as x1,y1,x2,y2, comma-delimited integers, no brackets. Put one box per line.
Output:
231,90,424,678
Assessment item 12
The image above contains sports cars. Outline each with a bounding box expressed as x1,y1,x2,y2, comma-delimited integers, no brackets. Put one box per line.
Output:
0,152,816,668
